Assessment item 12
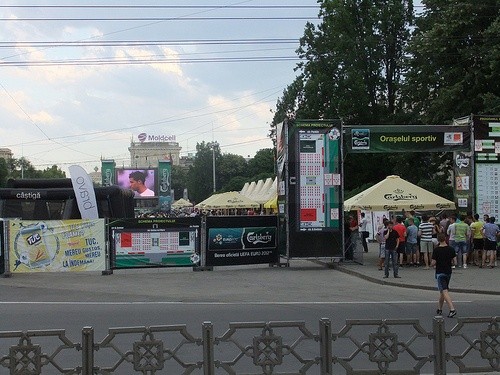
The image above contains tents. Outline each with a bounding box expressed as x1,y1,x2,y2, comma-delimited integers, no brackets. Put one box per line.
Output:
344,175,456,211
171,177,277,207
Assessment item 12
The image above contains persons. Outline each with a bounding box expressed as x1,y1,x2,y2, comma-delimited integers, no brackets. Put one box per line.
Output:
128,171,154,196
136,207,278,218
431,232,457,318
349,211,500,278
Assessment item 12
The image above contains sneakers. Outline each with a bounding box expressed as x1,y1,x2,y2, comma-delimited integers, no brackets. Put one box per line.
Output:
447,309,457,318
436,309,443,315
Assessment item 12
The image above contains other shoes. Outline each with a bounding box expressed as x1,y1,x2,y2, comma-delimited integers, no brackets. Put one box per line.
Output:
377,262,433,270
394,274,402,278
383,275,388,278
458,259,497,269
451,265,456,268
